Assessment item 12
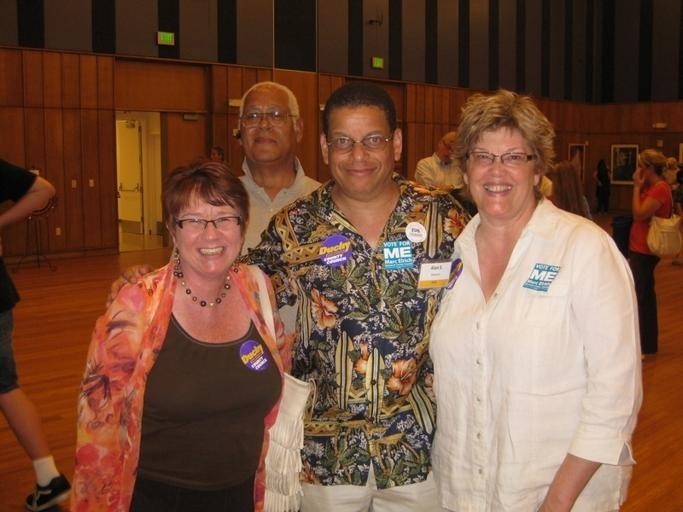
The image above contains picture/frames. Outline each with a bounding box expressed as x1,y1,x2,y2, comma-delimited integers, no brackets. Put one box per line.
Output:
610,144,639,185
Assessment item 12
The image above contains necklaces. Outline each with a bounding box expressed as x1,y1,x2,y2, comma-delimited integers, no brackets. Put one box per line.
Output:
172,254,236,309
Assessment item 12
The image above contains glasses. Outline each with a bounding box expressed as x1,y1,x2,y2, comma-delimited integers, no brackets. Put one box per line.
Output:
326,133,393,155
239,105,296,128
472,151,537,169
173,216,243,234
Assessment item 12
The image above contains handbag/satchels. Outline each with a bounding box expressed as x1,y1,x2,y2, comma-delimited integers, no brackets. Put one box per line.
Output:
647,214,682,259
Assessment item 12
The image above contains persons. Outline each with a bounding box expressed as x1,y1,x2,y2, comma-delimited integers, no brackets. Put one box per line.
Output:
426,86,644,512
413,131,466,204
237,81,324,254
0,159,72,510
545,119,683,359
238,85,472,512
71,157,295,511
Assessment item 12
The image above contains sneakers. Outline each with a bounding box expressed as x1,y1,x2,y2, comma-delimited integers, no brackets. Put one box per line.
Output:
25,473,73,511
